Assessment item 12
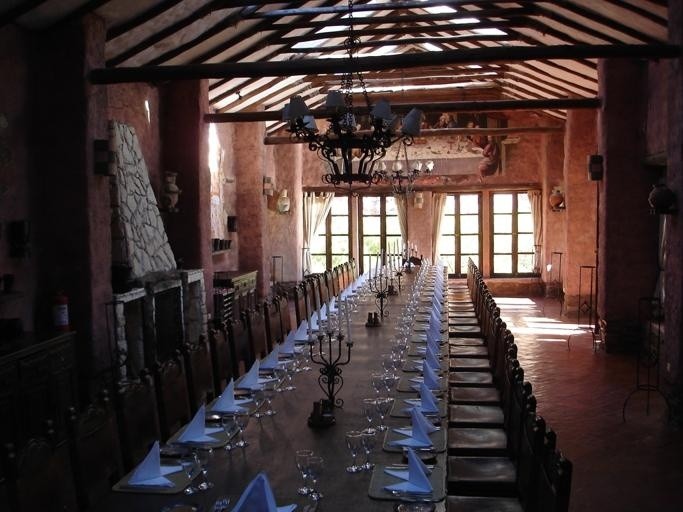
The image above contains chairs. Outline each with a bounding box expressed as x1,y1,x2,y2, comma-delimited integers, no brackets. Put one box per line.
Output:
336,265,344,292
265,295,283,353
445,257,575,512
0,367,164,511
317,274,329,305
330,267,339,296
346,261,353,284
150,348,194,435
181,333,214,414
341,263,348,289
247,302,269,366
278,294,297,336
349,258,357,282
293,283,307,328
228,310,251,378
312,275,322,311
208,311,235,393
303,277,314,319
325,268,334,301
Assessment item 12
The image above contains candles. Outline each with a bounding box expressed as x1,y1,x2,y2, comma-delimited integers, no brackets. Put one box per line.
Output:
338,290,343,334
306,294,313,344
397,239,400,272
374,251,379,277
369,254,371,279
400,243,407,259
315,285,324,336
345,295,351,343
411,242,414,257
393,241,396,270
385,248,388,277
388,242,393,275
324,286,332,331
380,249,383,274
407,239,410,261
415,244,417,259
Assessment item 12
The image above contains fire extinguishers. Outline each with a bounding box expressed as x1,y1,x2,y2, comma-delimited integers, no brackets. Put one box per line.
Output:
52,287,70,334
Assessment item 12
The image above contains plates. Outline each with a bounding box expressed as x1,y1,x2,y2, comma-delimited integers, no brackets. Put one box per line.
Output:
367,464,447,504
396,375,448,393
384,424,448,457
204,396,265,415
418,306,447,312
233,371,285,390
414,323,447,332
415,313,447,322
112,460,203,493
409,343,449,357
386,389,451,416
166,423,240,451
411,334,448,342
402,358,449,373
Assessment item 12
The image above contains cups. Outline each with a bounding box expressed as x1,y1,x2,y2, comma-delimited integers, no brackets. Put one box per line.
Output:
371,372,383,398
180,453,196,497
384,371,395,398
237,412,249,447
376,398,387,422
393,351,400,372
343,429,364,476
381,352,395,375
221,415,236,451
364,398,376,424
196,447,215,491
307,457,324,501
264,382,276,416
273,364,284,392
295,449,313,494
253,389,265,420
360,426,380,474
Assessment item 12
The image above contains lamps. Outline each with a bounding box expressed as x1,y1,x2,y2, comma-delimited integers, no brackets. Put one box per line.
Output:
377,138,436,194
278,0,429,185
